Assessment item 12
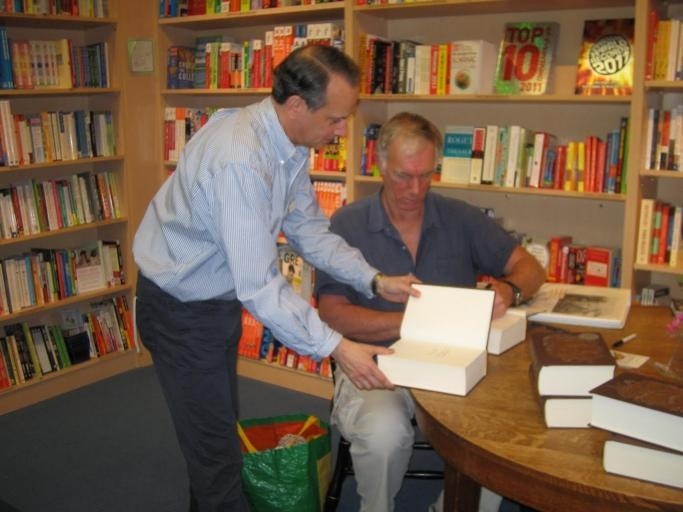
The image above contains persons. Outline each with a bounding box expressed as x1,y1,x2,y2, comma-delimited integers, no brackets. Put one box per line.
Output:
130,43,421,512
311,111,545,512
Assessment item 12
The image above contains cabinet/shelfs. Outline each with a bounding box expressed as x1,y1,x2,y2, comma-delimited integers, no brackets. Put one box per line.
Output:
152,0,351,407
0,2,151,422
350,2,645,303
621,2,682,310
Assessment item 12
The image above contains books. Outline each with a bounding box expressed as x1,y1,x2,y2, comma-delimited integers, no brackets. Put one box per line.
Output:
522,282,633,331
374,281,496,396
587,372,683,454
0,0,347,394
485,305,547,357
602,432,683,491
527,364,596,432
355,1,683,309
527,326,618,396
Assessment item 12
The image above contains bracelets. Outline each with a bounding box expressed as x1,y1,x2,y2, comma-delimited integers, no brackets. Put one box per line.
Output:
501,280,523,307
371,273,384,297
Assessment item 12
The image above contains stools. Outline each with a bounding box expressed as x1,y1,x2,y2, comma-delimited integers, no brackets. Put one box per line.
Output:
320,346,445,512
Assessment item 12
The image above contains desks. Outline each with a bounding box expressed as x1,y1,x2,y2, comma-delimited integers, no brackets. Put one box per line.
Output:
410,298,682,512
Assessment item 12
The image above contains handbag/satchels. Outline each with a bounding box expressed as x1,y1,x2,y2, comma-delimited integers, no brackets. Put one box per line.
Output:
236,411,333,512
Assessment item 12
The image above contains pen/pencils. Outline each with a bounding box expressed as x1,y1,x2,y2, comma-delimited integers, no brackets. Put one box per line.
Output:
611,333,638,349
